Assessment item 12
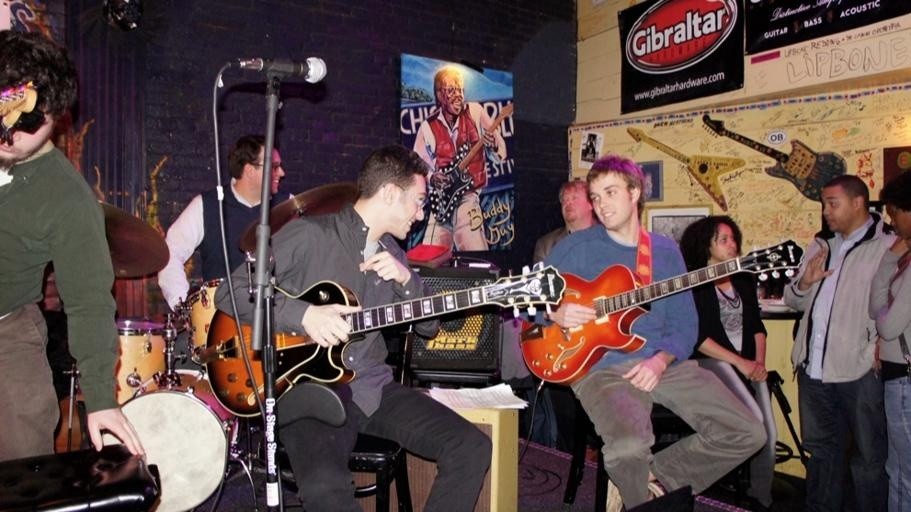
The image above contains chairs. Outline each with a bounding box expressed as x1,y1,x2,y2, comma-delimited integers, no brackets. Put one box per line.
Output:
242,319,423,511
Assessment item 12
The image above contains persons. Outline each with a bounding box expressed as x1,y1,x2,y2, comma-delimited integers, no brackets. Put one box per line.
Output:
519,154,766,511
158,137,288,313
671,219,776,511
409,65,510,253
869,166,911,511
782,172,893,511
0,31,147,464
214,146,494,512
532,178,604,270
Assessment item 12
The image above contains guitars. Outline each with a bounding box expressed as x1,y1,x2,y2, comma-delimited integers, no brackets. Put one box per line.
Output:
204,261,567,417
701,114,846,201
428,104,514,225
520,239,806,384
626,127,746,211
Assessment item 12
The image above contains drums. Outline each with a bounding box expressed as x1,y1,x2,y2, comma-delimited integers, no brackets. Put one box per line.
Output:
103,369,243,511
117,319,176,407
174,277,227,366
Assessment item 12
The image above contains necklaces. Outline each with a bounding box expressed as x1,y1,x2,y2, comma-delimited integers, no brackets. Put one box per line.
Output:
717,285,742,309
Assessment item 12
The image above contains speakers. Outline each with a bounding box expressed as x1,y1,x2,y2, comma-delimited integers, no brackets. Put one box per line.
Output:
410,269,501,383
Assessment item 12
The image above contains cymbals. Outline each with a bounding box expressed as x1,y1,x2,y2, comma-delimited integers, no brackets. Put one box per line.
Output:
99,200,169,278
238,183,362,252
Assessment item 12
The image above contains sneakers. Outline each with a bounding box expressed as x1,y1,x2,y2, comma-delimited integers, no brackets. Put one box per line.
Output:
605,476,623,512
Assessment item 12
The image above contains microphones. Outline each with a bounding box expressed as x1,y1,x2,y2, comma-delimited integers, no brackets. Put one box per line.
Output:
232,56,327,84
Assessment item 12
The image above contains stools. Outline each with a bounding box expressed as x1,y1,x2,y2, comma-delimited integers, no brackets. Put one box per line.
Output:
543,379,711,511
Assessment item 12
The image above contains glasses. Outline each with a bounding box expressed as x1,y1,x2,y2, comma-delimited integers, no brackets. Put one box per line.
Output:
383,181,429,210
252,160,285,172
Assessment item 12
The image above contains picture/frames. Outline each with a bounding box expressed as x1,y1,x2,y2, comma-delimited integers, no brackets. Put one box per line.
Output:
643,202,716,246
